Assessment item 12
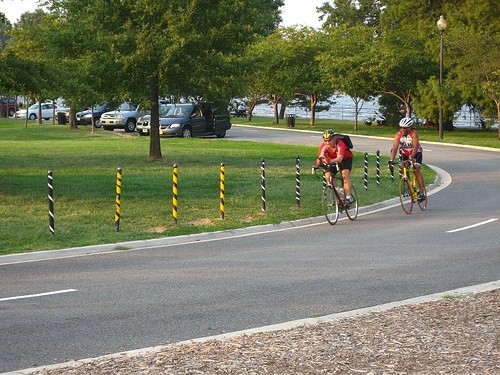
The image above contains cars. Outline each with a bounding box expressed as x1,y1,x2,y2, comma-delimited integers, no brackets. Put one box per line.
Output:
13,102,71,121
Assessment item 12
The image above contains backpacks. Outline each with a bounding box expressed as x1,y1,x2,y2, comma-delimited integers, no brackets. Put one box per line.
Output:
332,134,353,148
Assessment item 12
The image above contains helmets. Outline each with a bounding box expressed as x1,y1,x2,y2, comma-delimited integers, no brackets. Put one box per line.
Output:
399,117,414,127
322,129,334,140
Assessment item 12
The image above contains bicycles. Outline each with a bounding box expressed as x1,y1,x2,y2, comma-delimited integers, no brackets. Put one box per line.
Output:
313,161,359,225
389,160,428,215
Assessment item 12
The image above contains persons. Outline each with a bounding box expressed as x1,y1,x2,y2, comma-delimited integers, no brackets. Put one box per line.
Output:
238,104,244,111
389,117,425,201
315,129,353,204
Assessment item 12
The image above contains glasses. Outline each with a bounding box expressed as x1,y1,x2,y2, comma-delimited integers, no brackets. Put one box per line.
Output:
400,127,410,129
324,138,333,142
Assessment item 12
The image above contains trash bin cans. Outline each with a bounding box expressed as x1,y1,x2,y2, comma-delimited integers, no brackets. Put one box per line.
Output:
285,114,296,127
57,112,66,125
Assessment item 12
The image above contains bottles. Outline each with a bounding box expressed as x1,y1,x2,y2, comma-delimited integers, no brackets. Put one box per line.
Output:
410,180,416,192
335,186,345,201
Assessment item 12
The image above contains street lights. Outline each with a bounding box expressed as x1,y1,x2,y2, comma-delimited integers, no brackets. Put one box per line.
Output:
436,14,447,141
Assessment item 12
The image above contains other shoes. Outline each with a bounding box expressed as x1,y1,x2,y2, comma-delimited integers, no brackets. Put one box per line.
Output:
345,197,351,207
338,203,343,212
402,192,410,197
418,194,424,200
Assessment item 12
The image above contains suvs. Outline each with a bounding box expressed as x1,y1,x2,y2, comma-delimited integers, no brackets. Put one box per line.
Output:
0,96,19,118
160,101,231,138
75,97,176,135
